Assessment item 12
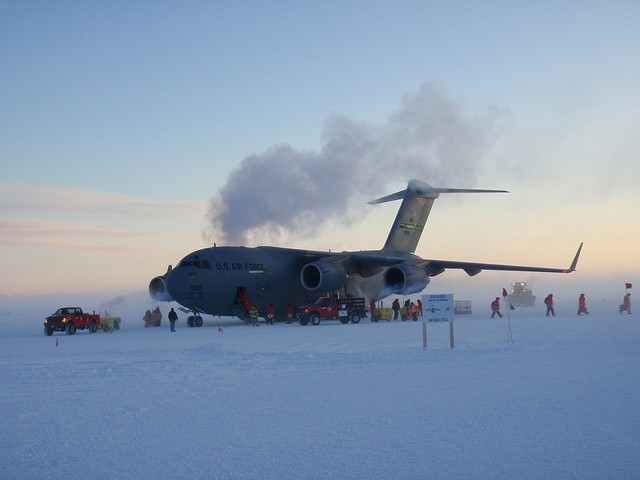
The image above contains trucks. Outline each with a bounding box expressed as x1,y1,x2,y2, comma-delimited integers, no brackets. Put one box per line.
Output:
297,297,369,325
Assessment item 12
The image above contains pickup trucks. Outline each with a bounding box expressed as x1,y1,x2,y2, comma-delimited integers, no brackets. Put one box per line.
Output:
44,307,100,335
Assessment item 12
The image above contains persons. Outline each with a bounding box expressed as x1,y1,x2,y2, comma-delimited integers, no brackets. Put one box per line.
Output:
266,304,273,325
370,298,376,321
249,305,260,327
417,300,422,318
286,304,294,324
392,298,400,320
619,293,633,315
491,297,503,319
410,301,418,321
576,293,589,316
544,294,555,316
168,308,178,331
405,299,410,305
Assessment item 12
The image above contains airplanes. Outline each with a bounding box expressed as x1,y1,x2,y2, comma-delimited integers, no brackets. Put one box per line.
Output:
149,180,583,327
